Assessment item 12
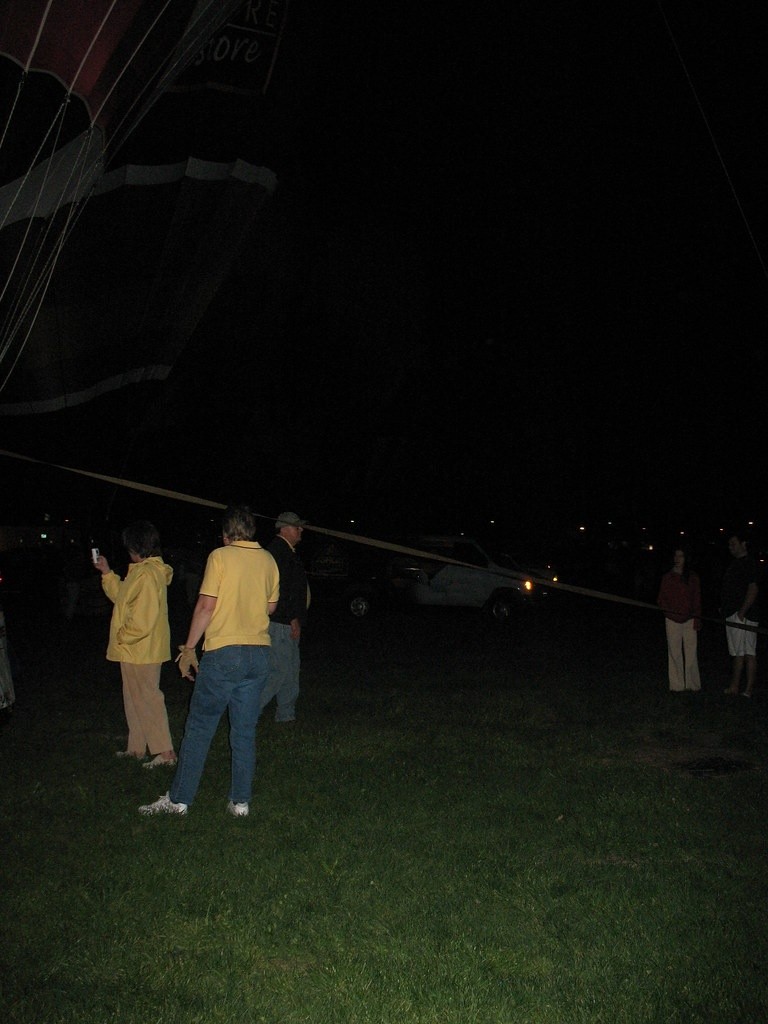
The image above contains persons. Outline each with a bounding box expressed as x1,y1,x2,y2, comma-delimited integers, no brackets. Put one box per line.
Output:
92,525,179,768
137,506,280,819
719,532,762,698
658,547,703,700
258,511,313,741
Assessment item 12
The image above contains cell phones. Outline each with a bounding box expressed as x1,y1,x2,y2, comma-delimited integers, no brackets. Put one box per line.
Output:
92,548,99,564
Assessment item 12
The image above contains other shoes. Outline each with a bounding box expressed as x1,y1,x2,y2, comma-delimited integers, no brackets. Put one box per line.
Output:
141,754,178,768
115,750,130,757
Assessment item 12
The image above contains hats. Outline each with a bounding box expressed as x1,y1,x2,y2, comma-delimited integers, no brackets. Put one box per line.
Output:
275,512,308,528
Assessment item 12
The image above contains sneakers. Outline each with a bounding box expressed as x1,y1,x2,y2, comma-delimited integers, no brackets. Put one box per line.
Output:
228,801,249,816
138,791,188,814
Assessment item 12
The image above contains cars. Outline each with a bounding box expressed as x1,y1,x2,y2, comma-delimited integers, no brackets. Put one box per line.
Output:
404,534,561,625
306,567,387,620
